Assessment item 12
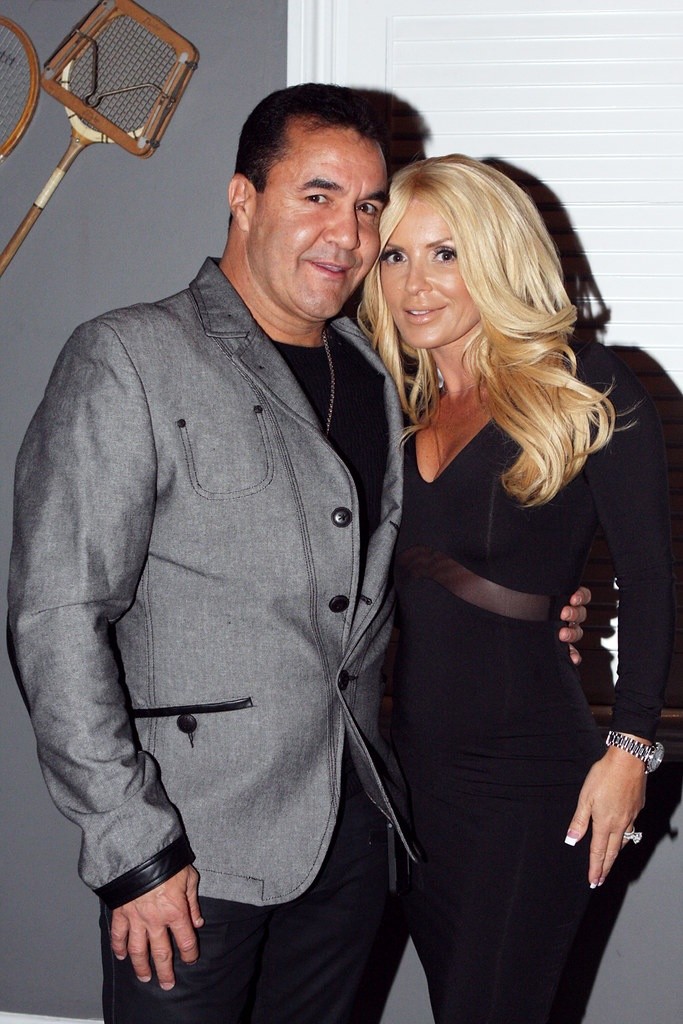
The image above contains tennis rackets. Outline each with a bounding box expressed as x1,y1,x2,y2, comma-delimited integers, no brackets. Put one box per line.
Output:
0,15,40,164
0,9,177,278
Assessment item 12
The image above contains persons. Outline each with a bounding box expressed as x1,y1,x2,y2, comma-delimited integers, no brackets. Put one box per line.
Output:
356,153,683,1024
5,83,589,1024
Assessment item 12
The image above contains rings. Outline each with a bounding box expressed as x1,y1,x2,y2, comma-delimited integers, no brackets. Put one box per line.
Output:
623,826,642,844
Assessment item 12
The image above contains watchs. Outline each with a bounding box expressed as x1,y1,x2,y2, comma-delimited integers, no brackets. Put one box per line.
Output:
605,731,664,775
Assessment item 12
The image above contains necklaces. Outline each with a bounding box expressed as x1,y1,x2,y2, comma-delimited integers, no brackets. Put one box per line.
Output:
321,329,336,438
442,379,483,393
